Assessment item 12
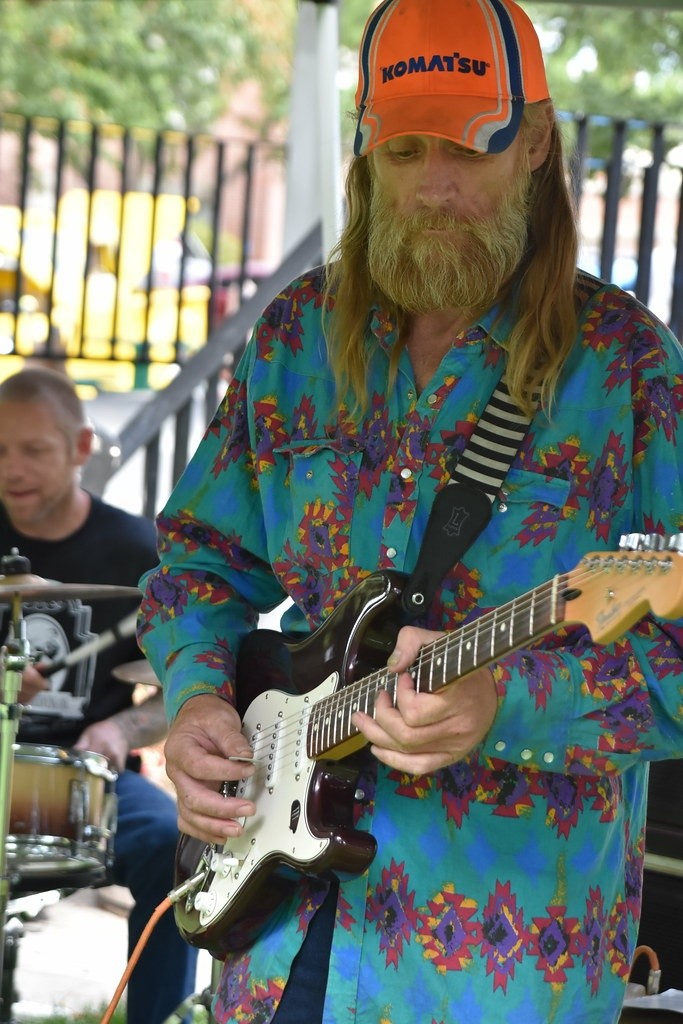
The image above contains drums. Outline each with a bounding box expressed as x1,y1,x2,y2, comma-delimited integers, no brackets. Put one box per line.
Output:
4,741,120,882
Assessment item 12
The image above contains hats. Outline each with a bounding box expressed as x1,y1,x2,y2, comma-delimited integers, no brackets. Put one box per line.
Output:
354,0,550,158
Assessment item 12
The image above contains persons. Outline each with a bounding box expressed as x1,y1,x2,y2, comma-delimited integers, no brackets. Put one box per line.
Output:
134,0,683,1024
1,365,199,1024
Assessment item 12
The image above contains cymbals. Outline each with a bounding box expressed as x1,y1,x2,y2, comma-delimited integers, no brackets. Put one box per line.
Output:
113,658,161,686
0,574,146,600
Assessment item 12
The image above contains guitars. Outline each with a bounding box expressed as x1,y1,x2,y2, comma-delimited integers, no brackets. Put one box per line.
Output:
172,528,683,957
